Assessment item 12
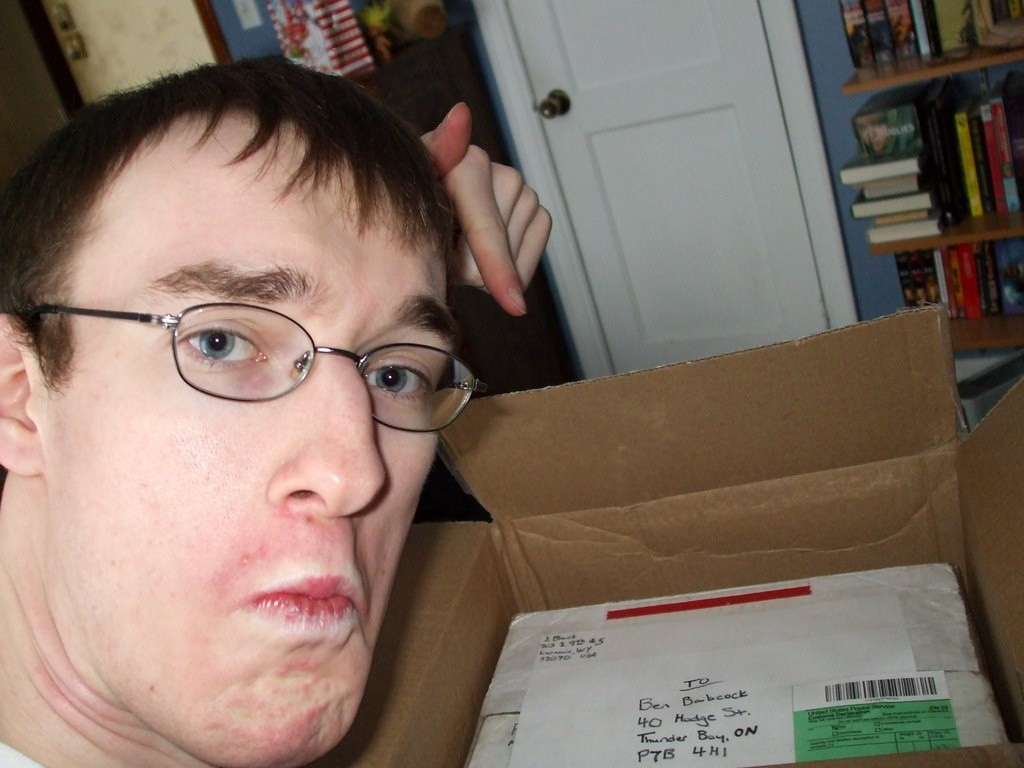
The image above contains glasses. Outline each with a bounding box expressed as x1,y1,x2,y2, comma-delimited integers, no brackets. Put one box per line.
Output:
16,302,487,433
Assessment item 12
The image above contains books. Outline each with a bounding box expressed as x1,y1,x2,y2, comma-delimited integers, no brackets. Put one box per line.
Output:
828,1,1024,354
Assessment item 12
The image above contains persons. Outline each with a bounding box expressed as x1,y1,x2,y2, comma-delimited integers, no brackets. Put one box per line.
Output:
3,52,551,764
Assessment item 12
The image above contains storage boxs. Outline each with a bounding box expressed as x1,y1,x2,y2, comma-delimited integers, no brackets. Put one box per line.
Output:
305,305,1024,768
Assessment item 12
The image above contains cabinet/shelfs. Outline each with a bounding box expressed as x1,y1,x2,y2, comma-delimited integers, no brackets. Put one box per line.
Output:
841,44,1024,351
